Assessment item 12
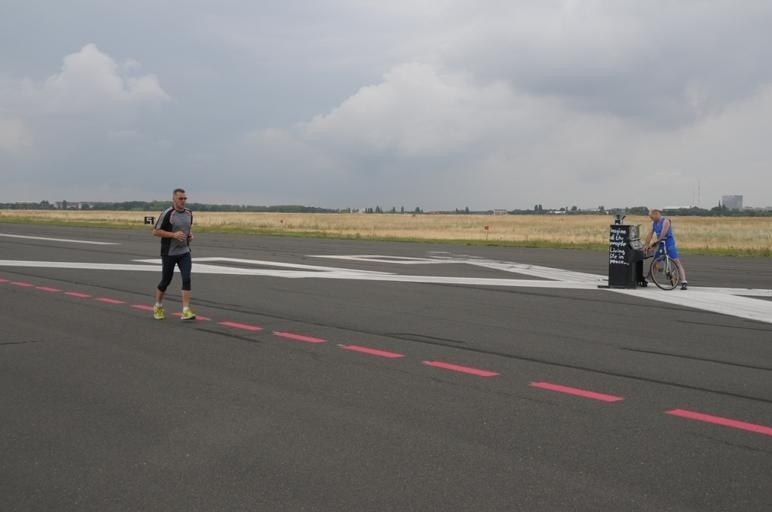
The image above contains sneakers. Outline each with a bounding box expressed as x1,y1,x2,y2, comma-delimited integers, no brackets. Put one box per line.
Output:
180,308,196,320
152,304,165,320
680,280,688,290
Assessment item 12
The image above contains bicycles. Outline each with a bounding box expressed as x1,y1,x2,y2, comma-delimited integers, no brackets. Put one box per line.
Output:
642,232,681,291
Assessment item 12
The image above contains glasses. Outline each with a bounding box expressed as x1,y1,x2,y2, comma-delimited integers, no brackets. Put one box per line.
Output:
177,197,188,201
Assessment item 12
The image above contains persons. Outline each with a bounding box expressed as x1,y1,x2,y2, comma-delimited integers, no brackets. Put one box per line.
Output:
152,188,197,321
640,208,688,290
